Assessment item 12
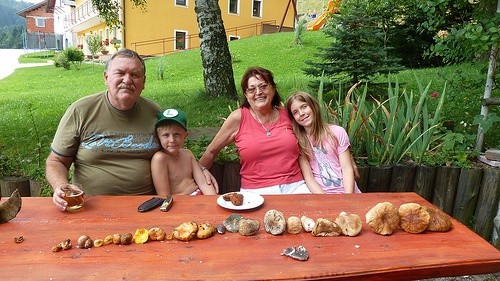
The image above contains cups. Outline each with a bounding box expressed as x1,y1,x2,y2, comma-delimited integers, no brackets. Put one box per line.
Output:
61,184,84,211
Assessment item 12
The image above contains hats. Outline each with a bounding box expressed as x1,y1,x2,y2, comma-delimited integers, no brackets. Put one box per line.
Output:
154,109,187,130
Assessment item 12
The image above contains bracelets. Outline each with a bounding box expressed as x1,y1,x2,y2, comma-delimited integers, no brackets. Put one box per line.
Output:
202,166,207,171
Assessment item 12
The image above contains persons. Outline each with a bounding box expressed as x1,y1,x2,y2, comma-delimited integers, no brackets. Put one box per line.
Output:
200,67,313,194
285,91,363,194
46,48,219,212
150,108,217,196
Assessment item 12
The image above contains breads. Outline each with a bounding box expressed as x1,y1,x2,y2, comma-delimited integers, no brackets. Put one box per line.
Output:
14,236,24,243
222,213,244,232
222,193,244,206
335,211,362,236
301,216,316,231
263,209,287,235
421,206,453,232
196,223,215,238
52,227,173,252
216,224,226,234
366,201,400,236
173,221,197,240
312,218,342,237
238,218,260,235
287,216,302,234
397,202,430,234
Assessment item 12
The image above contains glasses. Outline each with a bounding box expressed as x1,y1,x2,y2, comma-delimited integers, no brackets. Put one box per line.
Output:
246,82,271,93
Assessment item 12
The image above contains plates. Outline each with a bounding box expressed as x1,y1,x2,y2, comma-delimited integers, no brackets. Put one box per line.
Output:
216,191,264,209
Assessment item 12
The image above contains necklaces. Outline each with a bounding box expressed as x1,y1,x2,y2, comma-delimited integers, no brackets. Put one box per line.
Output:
253,112,273,136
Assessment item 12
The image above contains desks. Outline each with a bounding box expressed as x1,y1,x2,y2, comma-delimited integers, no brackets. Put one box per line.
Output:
0,193,500,281
